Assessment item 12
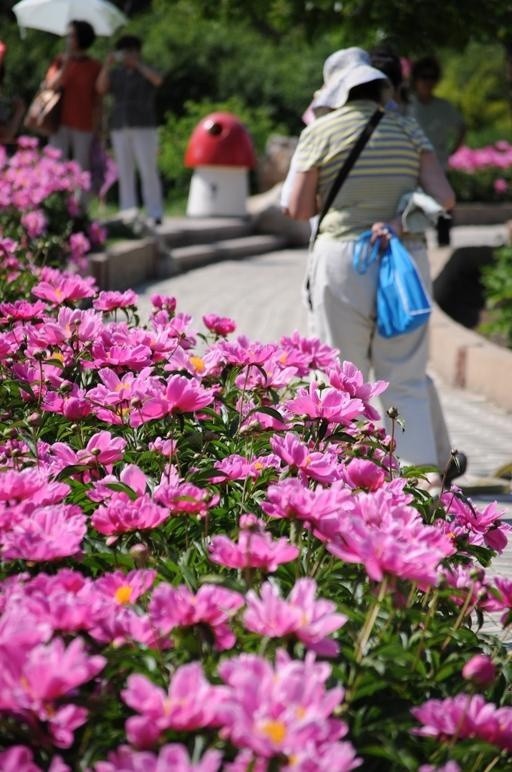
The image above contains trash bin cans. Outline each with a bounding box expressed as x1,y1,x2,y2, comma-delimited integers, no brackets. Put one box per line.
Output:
184,112,256,218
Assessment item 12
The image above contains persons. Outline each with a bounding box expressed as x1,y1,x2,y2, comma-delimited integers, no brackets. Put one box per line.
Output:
301,41,403,122
39,21,106,178
397,56,470,169
93,33,169,231
281,42,455,479
0,40,28,146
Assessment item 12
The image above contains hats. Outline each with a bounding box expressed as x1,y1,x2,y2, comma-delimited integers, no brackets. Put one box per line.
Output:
310,46,392,119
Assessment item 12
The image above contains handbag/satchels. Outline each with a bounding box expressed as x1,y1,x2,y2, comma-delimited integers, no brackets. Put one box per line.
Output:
23,88,66,134
352,224,432,340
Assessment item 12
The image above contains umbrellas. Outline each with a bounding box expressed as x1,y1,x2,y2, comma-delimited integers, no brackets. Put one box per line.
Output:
11,1,126,39
